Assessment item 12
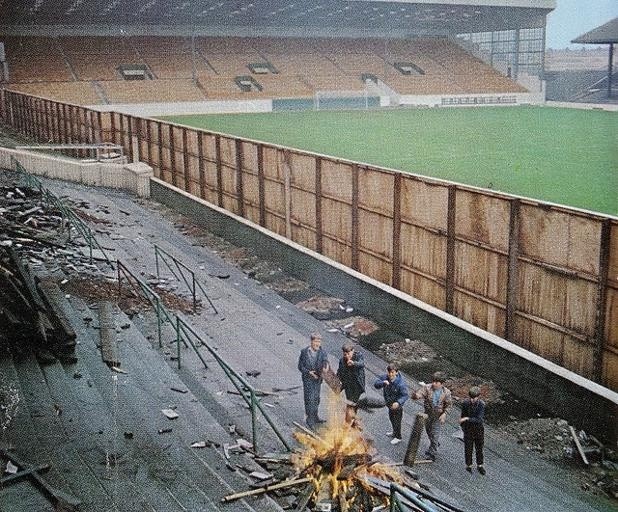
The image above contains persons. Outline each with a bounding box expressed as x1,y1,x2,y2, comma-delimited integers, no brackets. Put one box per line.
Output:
410,370,453,457
374,362,409,445
458,386,487,476
335,341,366,433
298,332,330,432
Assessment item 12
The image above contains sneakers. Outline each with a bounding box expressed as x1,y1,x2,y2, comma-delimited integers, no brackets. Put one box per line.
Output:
425,449,436,462
477,466,486,475
466,465,472,471
306,416,328,426
385,432,402,445
